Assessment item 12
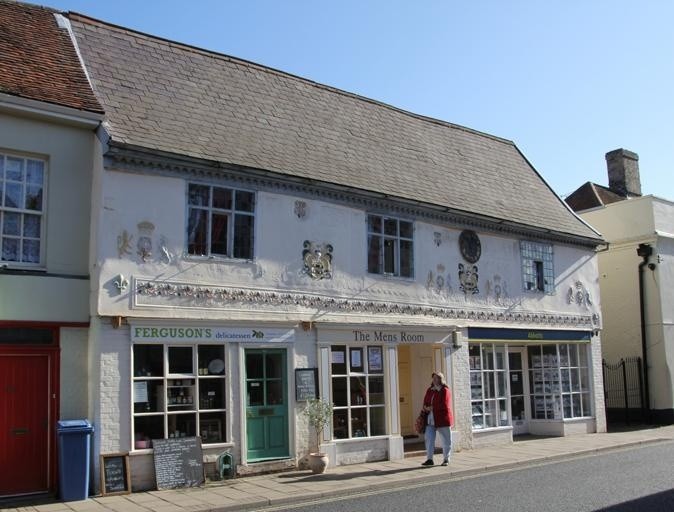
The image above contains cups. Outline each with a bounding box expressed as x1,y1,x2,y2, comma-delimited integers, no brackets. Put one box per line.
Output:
199,368,208,375
354,394,364,405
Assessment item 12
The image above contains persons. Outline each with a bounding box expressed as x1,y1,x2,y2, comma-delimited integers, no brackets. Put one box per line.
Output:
350,376,366,430
421,371,454,466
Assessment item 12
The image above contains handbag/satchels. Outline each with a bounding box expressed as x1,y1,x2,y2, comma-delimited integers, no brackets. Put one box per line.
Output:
414,410,428,435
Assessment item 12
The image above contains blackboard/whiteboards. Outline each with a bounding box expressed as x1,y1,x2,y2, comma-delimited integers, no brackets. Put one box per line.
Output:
152,436,204,491
294,368,320,402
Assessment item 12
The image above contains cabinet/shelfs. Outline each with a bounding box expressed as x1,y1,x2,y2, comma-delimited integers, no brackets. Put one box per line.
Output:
132,343,233,448
469,355,495,430
531,354,579,419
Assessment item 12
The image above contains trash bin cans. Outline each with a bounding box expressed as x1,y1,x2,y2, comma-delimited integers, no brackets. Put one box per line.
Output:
56,419,95,502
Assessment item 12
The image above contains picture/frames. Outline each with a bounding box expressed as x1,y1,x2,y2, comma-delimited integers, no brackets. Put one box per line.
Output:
331,345,384,374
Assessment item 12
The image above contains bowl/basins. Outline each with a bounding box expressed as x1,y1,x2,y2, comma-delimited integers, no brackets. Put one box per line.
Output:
136,438,150,449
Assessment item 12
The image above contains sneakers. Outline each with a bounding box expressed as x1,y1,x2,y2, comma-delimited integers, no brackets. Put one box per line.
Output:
421,459,449,466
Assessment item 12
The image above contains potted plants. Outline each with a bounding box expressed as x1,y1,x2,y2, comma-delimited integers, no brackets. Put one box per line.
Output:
302,398,336,474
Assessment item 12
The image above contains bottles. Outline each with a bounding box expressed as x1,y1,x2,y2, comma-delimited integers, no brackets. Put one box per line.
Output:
145,401,150,413
200,392,214,409
340,417,367,438
167,379,194,405
169,431,187,438
208,359,225,373
